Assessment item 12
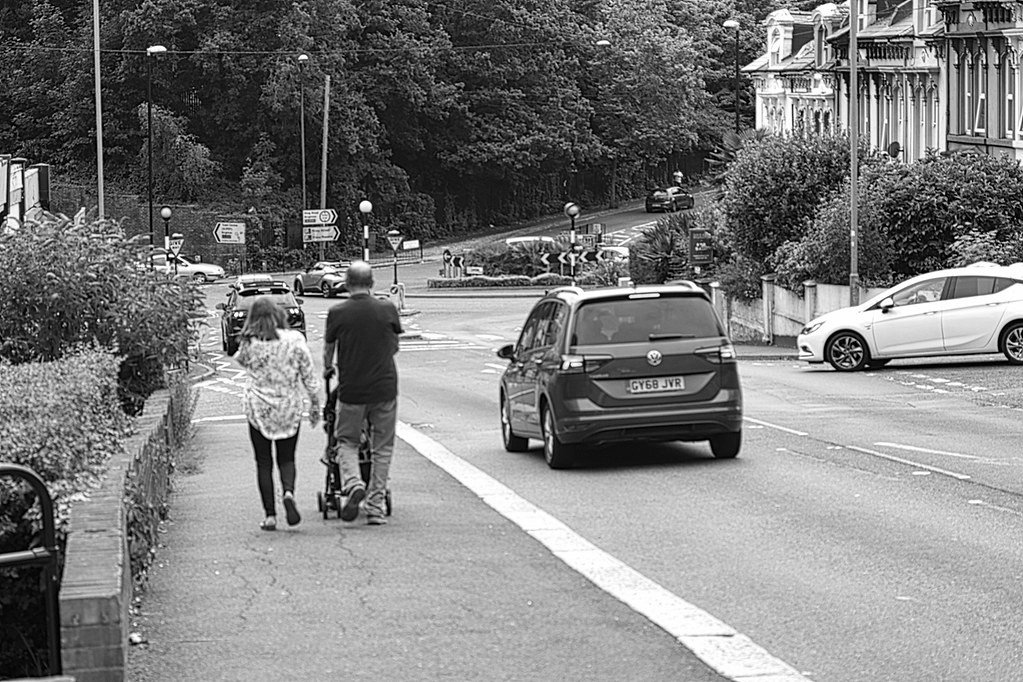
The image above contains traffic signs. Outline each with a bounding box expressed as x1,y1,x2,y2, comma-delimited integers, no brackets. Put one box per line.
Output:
212,222,246,244
303,226,340,242
302,209,338,225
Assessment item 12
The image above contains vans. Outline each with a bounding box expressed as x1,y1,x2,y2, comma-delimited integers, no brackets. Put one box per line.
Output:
504,235,563,255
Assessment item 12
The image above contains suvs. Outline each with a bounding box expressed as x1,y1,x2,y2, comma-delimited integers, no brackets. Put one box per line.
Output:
645,185,695,213
498,278,744,469
293,260,353,299
216,288,307,356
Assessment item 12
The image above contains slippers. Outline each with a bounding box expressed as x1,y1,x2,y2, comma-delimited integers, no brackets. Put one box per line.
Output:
284,495,300,526
259,521,276,530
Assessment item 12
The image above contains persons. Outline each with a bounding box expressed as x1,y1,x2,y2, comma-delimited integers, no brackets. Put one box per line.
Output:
235,298,319,530
669,165,688,187
323,261,404,525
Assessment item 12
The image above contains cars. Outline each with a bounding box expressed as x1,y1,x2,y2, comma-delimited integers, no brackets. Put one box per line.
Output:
142,253,225,285
225,278,293,293
797,266,1023,373
233,274,273,285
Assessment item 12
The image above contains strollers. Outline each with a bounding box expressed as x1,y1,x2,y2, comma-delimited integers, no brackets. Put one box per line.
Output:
317,367,393,520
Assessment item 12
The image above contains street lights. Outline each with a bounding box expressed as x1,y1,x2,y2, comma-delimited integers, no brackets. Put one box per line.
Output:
297,53,310,211
563,202,580,278
722,19,740,134
161,207,172,251
145,45,168,254
387,229,404,284
359,200,373,264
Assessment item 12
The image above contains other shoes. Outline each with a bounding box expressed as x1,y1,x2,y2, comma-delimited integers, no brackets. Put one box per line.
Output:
342,485,365,522
367,515,388,525
